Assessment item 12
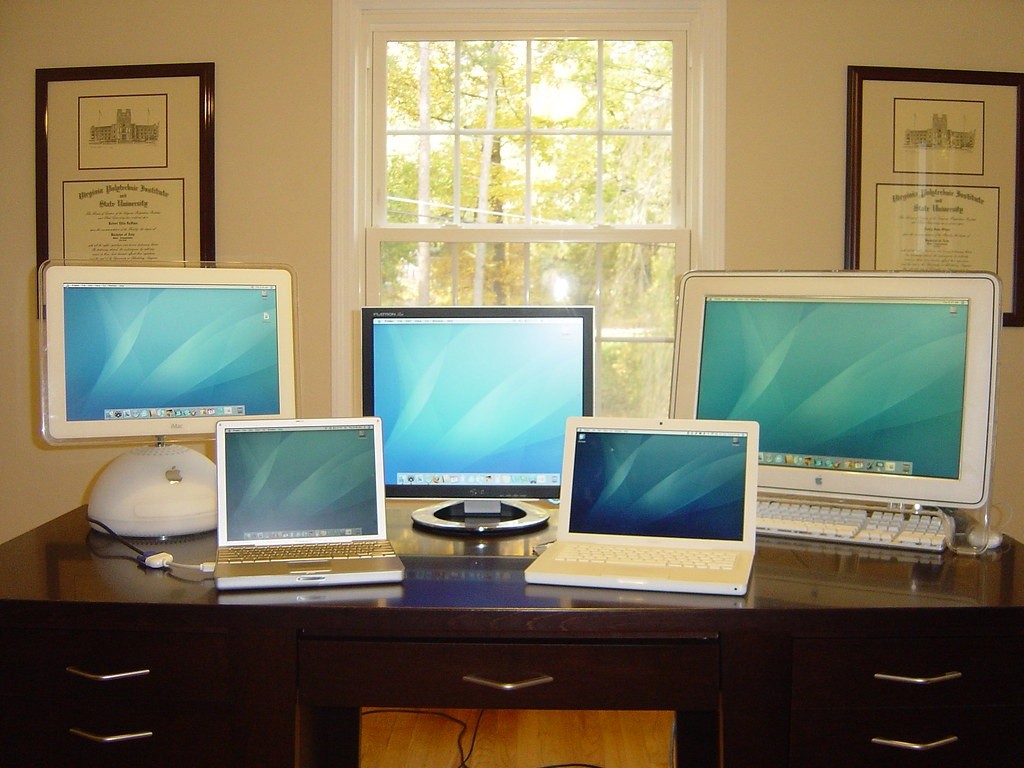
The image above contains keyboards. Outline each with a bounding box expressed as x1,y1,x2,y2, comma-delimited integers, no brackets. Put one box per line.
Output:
754,501,954,552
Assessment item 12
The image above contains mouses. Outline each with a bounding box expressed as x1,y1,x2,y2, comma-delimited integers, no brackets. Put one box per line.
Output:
968,523,1004,549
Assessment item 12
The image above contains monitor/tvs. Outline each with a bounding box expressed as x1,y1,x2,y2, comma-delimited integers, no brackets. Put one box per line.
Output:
668,270,1003,508
361,306,595,531
38,260,300,538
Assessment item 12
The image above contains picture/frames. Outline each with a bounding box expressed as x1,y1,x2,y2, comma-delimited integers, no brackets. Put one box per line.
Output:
36,62,216,320
844,66,1024,329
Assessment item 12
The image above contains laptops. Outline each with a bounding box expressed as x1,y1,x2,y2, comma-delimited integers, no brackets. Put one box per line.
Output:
523,415,759,595
215,417,406,590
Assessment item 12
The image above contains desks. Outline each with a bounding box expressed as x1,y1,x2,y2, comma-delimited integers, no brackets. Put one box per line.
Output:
0,500,1024,768
